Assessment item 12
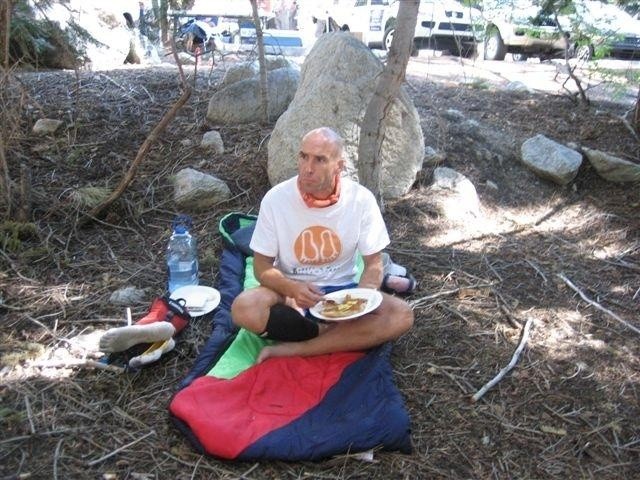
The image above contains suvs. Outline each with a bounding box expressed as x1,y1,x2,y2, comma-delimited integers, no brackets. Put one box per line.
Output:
340,0,483,58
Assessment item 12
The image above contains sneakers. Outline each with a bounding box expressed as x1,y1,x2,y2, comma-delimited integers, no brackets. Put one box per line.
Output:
98,321,175,367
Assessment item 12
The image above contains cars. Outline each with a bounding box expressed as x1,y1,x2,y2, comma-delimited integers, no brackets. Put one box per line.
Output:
484,0,573,58
570,2,640,60
167,13,303,58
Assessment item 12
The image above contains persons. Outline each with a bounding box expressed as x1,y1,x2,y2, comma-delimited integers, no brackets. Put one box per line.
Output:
231,126,415,364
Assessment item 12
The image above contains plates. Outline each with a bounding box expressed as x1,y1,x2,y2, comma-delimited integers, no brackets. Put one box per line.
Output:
169,285,222,318
309,288,382,322
